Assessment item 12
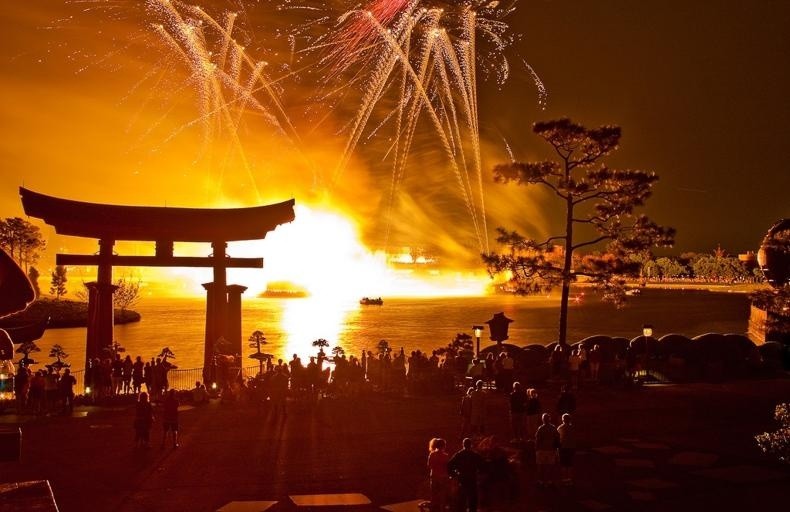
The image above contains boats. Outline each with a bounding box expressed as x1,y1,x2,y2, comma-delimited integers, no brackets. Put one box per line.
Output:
359,297,383,306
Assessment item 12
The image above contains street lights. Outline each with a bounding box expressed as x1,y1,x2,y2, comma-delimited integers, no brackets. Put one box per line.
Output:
474,324,484,360
639,326,655,375
